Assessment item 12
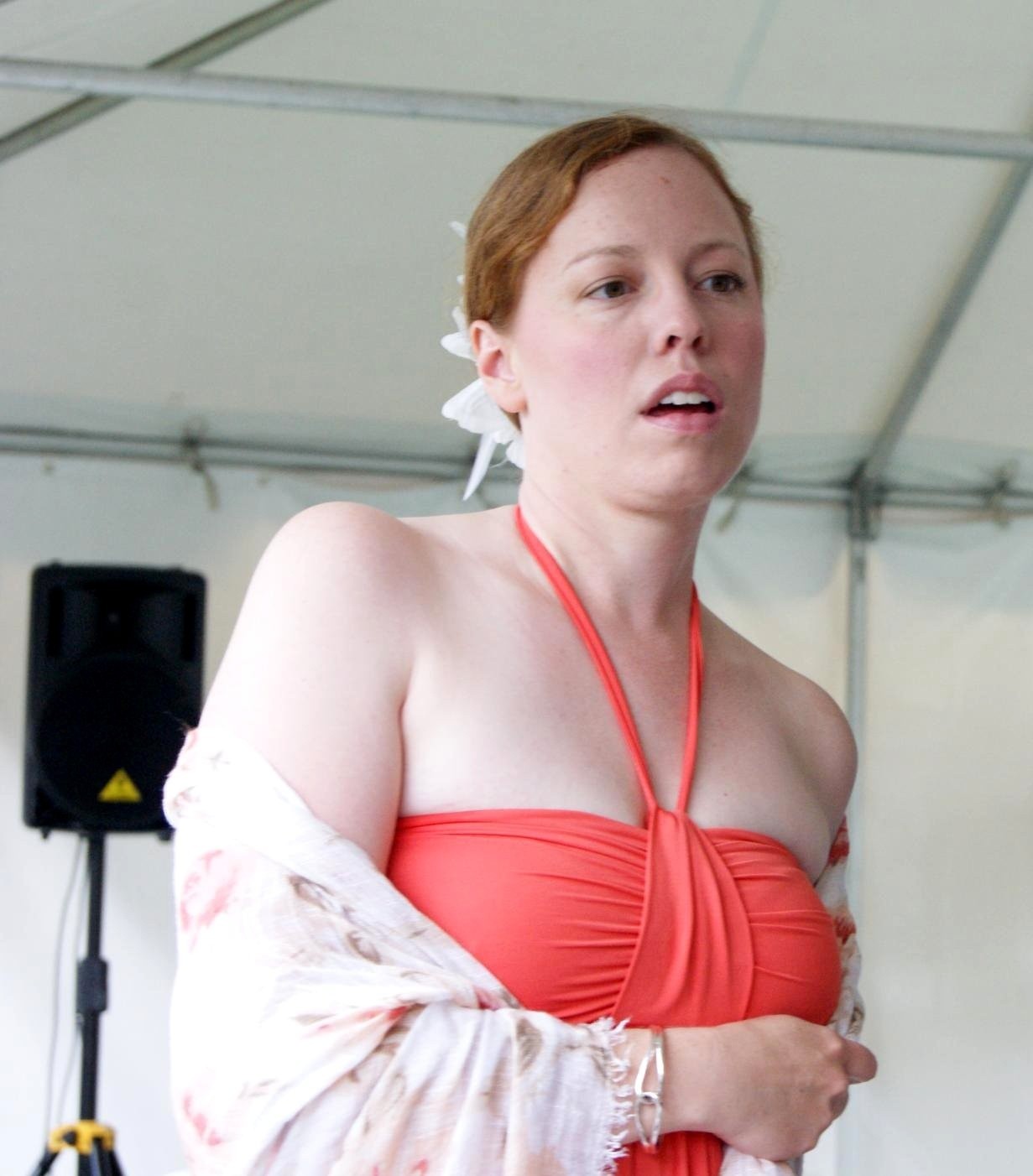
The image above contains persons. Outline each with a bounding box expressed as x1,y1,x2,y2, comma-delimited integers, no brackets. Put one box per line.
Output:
161,115,880,1176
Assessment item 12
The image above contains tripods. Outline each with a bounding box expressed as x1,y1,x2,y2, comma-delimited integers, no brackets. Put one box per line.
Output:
27,830,128,1176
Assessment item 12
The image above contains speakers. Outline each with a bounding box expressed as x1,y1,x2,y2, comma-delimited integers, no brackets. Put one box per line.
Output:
19,561,206,843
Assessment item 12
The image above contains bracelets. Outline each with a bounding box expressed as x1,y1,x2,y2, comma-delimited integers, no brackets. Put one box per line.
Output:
634,1026,666,1154
